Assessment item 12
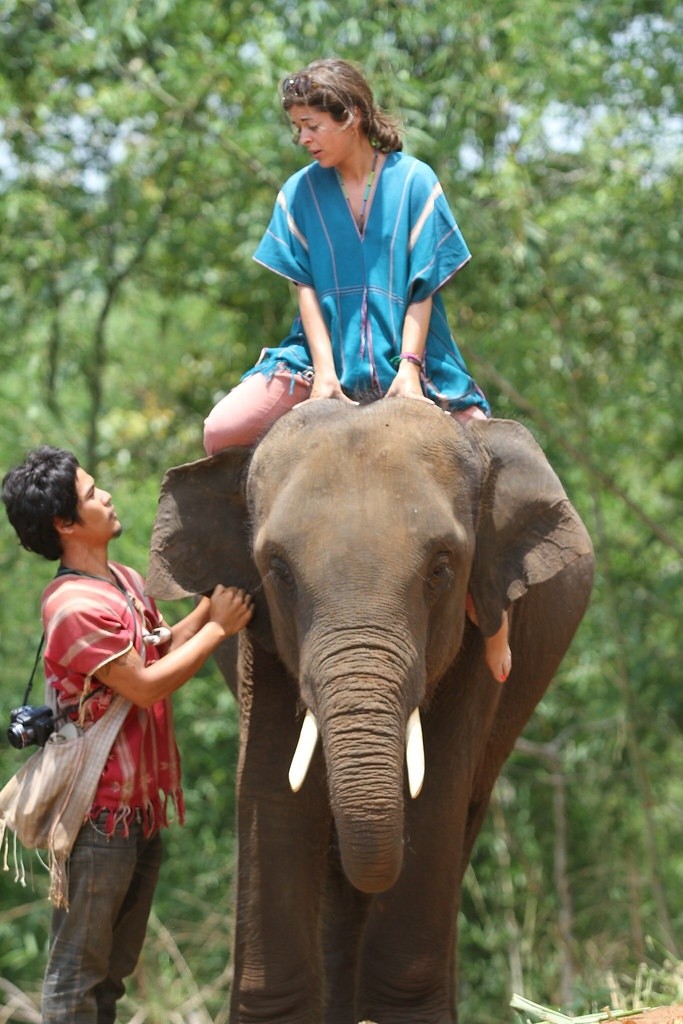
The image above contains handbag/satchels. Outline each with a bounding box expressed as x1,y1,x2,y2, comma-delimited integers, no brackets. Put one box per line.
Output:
0,726,114,912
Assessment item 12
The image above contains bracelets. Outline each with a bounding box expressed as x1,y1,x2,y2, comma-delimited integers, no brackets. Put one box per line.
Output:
388,351,425,369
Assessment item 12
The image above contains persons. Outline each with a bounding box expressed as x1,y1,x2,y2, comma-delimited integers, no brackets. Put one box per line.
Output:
0,447,255,1024
204,58,512,683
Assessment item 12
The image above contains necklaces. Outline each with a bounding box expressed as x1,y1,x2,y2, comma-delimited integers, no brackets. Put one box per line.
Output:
337,148,379,230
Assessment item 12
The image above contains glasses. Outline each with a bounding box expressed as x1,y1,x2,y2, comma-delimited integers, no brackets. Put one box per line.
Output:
281,73,334,97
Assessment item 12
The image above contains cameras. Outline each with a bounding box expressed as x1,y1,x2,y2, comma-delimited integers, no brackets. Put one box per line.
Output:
7,704,53,749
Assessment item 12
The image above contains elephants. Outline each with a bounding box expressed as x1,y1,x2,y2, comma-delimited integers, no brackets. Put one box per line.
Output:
149,398,593,1024
